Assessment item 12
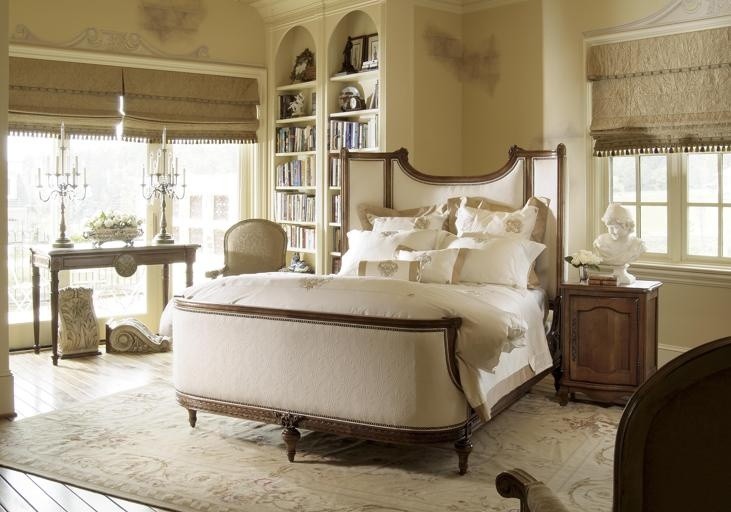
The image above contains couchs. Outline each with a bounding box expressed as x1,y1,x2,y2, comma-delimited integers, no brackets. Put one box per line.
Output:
204,219,287,279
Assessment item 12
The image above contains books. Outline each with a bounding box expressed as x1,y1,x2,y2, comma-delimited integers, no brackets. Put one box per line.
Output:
276,125,315,153
276,155,315,186
277,191,314,223
330,156,341,186
335,229,341,251
330,114,378,149
588,274,617,282
282,224,315,249
369,79,378,109
280,95,296,119
588,280,617,285
332,195,342,222
333,258,342,273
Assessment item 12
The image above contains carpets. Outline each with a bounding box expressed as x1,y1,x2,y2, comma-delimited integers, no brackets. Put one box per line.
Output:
0,380,624,510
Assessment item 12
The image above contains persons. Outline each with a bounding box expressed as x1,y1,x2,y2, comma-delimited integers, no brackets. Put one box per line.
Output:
592,203,647,265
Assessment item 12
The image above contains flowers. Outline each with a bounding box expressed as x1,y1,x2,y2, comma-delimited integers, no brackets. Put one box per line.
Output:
563,251,604,271
87,211,142,228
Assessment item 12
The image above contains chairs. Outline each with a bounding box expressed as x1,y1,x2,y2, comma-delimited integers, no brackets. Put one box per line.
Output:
496,334,731,512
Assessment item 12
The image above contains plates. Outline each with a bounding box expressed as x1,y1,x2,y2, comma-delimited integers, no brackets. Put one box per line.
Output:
338,87,361,110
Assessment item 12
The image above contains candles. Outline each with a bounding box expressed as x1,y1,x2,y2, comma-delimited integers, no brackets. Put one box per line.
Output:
156,149,161,174
162,128,166,148
149,153,154,174
168,152,171,172
172,164,174,173
141,164,145,184
75,155,78,173
72,166,76,185
182,168,185,185
84,167,87,186
60,120,65,147
174,157,178,175
66,150,71,173
55,153,58,173
47,155,50,173
37,167,41,185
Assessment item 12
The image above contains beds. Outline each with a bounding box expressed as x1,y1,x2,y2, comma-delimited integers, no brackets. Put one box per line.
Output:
172,143,567,474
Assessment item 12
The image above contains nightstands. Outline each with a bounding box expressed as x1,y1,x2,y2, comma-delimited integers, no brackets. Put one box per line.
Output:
559,281,662,406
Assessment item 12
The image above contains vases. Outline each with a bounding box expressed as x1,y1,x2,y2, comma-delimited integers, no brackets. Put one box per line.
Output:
578,265,589,283
90,228,137,249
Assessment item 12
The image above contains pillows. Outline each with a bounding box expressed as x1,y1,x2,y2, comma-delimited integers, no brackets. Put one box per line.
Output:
441,233,546,289
356,202,450,232
448,196,550,242
338,228,452,277
395,245,467,285
358,260,421,282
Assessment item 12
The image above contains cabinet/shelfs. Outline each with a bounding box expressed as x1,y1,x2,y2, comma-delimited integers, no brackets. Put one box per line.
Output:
251,0,322,273
29,244,201,366
323,1,389,274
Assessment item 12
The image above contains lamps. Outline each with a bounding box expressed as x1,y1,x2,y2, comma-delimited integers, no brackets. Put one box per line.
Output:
115,68,126,144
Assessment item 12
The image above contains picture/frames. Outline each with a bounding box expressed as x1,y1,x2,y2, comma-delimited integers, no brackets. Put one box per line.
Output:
350,37,365,72
367,33,378,60
